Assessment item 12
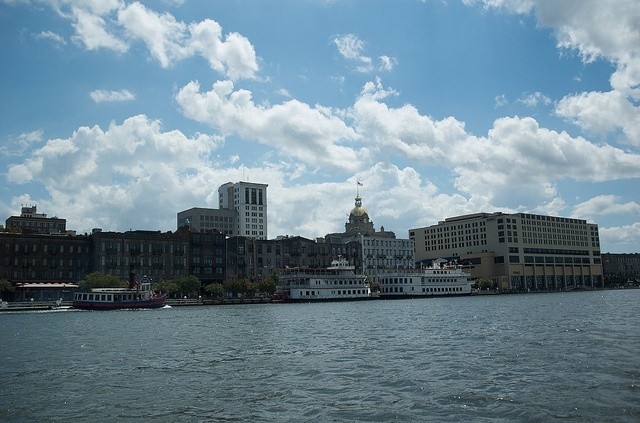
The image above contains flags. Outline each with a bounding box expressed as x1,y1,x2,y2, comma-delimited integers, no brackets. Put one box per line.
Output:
358,182,363,186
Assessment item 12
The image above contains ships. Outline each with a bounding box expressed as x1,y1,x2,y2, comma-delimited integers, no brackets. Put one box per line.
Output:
71,282,167,310
377,259,476,300
270,255,380,303
